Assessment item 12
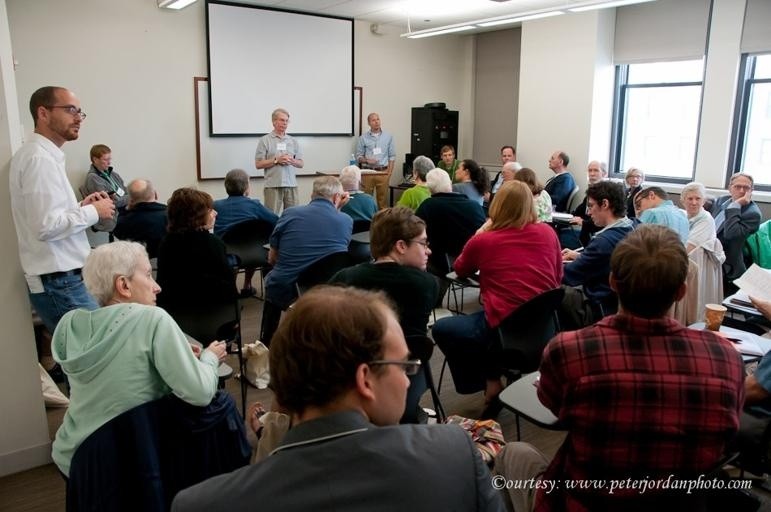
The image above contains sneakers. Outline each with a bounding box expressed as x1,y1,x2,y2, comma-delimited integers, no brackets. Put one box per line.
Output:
241,288,256,297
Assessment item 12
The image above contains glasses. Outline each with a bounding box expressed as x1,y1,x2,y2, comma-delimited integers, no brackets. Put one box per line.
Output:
52,106,86,119
368,359,422,376
407,239,430,249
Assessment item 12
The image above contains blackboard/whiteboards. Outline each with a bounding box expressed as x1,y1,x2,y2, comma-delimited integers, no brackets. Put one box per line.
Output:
194,77,362,182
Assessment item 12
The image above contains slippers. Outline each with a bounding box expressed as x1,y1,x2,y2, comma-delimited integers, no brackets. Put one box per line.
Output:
247,402,266,440
471,390,504,420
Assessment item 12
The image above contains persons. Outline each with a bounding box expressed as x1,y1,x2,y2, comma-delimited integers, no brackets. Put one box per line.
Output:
111,144,771,476
84,145,133,232
49,239,229,489
491,222,750,510
171,283,511,511
9,83,118,342
353,113,397,211
256,107,305,218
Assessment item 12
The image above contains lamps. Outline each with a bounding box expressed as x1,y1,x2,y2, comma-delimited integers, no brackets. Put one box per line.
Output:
157,0,196,11
399,0,649,40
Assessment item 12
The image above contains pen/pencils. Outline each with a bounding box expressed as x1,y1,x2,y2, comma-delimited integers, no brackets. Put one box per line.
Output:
725,337,743,343
342,197,354,199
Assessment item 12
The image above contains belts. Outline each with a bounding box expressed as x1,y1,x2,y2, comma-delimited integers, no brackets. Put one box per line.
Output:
360,166,387,171
40,269,81,281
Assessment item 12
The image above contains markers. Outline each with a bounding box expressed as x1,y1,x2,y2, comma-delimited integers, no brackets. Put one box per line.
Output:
316,172,324,175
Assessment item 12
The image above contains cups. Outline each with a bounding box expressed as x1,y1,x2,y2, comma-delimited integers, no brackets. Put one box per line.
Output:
704,303,728,332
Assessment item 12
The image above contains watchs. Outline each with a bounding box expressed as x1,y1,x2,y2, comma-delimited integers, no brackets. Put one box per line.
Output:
274,156,279,165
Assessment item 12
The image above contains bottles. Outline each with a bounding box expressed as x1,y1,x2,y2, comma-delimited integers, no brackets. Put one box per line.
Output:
350,154,356,166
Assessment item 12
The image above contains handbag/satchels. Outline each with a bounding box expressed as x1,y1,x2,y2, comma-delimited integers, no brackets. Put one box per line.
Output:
398,174,416,186
242,340,269,390
442,415,504,463
558,285,592,327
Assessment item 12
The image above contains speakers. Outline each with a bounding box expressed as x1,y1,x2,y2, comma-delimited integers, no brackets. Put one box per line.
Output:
412,107,458,167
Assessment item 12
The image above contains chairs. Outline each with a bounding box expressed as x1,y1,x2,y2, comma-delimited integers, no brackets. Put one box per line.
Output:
65,185,771,512
